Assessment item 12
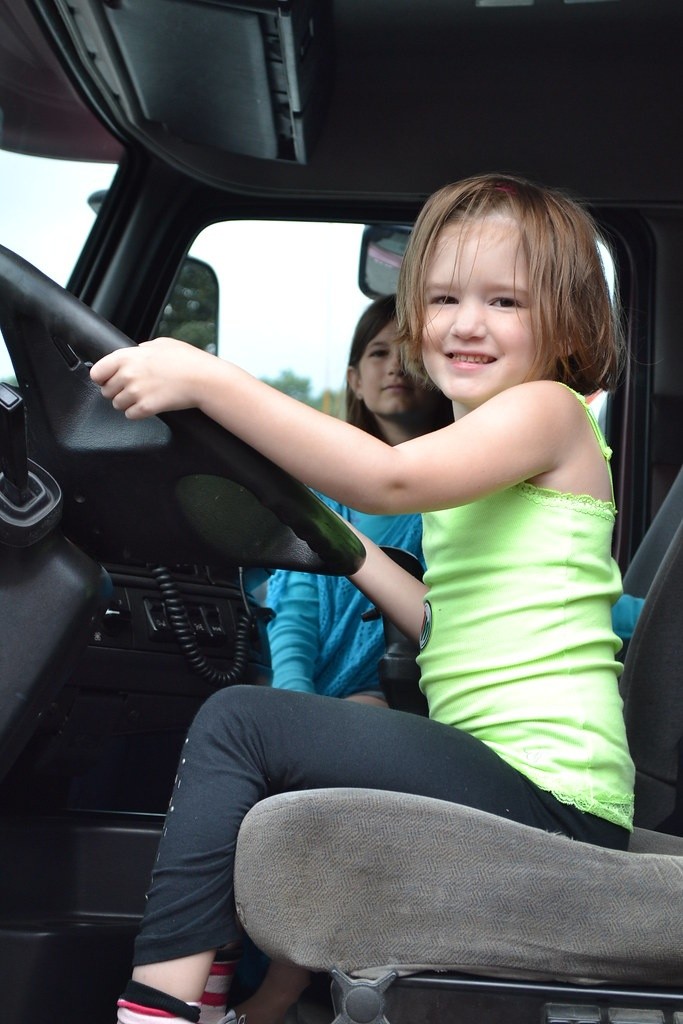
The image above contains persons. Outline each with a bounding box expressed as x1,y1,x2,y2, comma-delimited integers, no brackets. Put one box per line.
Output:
242,292,648,709
113,176,637,1024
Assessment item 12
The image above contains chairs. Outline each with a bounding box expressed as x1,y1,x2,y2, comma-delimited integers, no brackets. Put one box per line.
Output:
235,472,683,991
